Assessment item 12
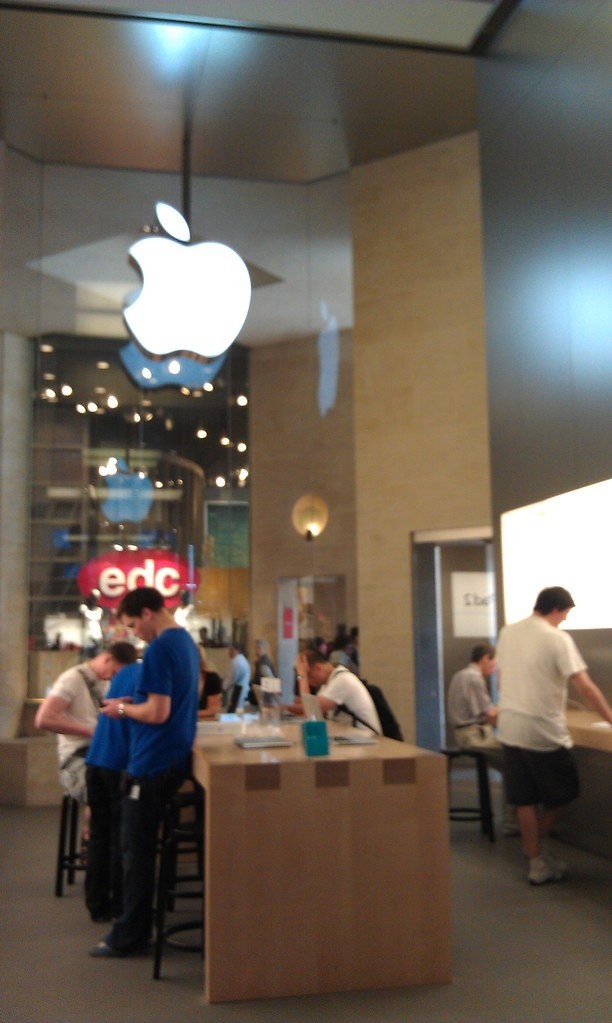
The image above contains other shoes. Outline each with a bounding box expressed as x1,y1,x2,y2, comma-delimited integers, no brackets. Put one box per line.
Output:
88,935,114,957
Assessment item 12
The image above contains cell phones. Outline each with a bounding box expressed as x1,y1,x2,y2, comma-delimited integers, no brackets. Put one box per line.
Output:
95,696,107,708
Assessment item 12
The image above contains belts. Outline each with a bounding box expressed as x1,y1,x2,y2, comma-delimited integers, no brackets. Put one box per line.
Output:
455,723,484,728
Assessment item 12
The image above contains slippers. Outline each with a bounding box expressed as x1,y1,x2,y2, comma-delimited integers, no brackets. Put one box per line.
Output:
527,869,564,884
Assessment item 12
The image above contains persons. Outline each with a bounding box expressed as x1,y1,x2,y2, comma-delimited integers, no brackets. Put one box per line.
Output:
34,586,276,957
447,642,519,835
494,587,612,886
265,623,385,738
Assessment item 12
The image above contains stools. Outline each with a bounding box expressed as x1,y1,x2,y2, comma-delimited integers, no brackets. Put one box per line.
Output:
137,787,205,980
439,747,498,843
54,789,91,898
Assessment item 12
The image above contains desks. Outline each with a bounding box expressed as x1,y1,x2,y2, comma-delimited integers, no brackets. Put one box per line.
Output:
189,704,453,1002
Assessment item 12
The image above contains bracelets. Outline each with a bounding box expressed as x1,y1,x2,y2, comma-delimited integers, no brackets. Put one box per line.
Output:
118,702,126,718
297,674,307,680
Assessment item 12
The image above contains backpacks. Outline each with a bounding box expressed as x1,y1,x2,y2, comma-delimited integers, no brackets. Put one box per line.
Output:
337,678,405,742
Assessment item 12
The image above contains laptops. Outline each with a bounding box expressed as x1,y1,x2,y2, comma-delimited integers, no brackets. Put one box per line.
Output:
235,685,379,749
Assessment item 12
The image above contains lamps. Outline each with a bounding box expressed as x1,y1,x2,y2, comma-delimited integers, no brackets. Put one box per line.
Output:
291,489,329,539
